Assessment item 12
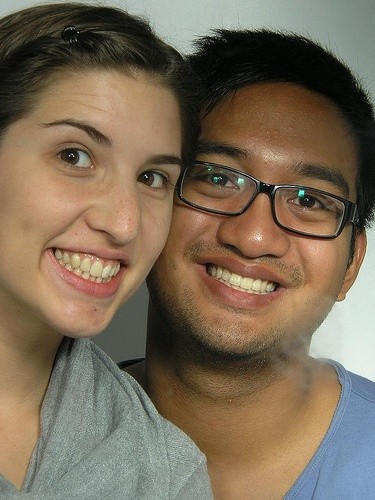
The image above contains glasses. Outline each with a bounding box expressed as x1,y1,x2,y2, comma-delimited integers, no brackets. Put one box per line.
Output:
176,159,358,240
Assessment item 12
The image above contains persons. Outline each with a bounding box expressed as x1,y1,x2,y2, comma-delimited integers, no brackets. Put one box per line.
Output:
108,26,375,500
1,0,215,499
